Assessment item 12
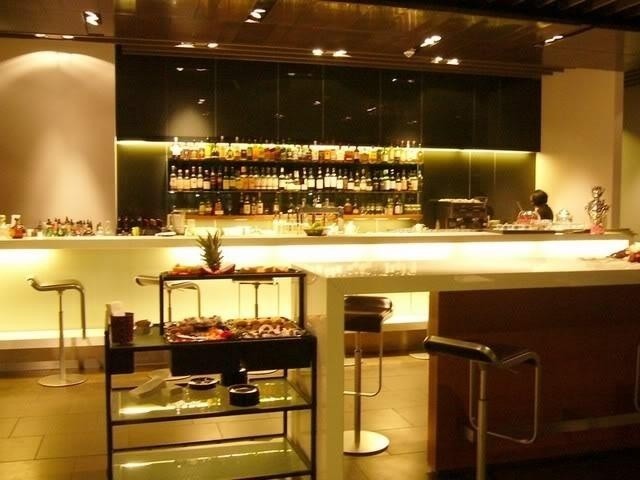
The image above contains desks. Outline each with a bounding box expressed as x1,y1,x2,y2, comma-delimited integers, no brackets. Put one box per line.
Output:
291,238,639,480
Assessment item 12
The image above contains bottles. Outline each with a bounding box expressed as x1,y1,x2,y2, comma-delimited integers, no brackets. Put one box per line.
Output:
330,168,337,189
315,198,322,208
401,176,407,191
197,166,203,190
230,166,235,191
354,179,360,191
247,139,252,161
379,177,385,191
256,200,263,214
191,166,197,191
240,139,246,161
294,170,300,192
312,141,319,161
205,136,211,160
242,200,250,215
337,175,344,190
336,140,423,164
223,166,230,191
279,167,284,190
330,149,336,161
347,177,355,190
203,170,211,191
216,168,222,190
264,136,270,161
250,203,256,215
271,167,279,191
241,166,248,191
344,201,352,214
372,177,379,191
287,174,294,192
300,168,308,190
183,170,190,191
272,198,279,215
303,144,312,160
211,141,219,160
176,169,183,191
225,140,235,161
394,197,403,215
343,176,348,189
234,136,240,161
324,167,331,189
37,217,103,237
301,144,304,152
211,169,216,190
365,178,373,192
390,170,395,190
249,166,254,191
395,173,401,191
218,135,225,160
280,137,286,160
384,176,390,191
254,167,261,190
360,175,366,191
275,137,280,161
297,147,302,160
316,167,324,189
293,140,297,160
252,139,258,161
266,168,272,191
308,168,315,190
9,218,27,239
352,202,360,215
235,171,240,189
270,138,276,161
189,138,198,160
198,201,205,216
286,137,293,160
182,140,189,160
206,200,211,215
260,167,266,190
170,137,182,160
215,202,222,216
198,137,205,160
258,137,264,162
169,165,176,191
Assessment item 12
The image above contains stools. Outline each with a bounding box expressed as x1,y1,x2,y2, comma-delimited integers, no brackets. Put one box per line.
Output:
423,336,540,480
135,275,202,323
28,278,89,387
238,280,281,319
344,294,391,456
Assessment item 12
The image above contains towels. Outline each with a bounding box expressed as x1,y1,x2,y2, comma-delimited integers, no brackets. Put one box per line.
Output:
159,382,183,396
129,375,167,399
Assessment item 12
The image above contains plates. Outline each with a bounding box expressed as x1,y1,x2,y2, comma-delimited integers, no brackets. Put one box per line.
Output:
156,232,179,237
188,377,219,385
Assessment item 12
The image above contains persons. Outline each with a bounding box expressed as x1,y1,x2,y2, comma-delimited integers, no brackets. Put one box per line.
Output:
529,190,553,221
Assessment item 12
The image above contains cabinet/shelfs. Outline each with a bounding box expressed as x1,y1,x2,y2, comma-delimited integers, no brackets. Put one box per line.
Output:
116,38,215,144
116,147,536,237
104,268,318,480
323,51,420,148
421,57,541,154
216,44,322,145
